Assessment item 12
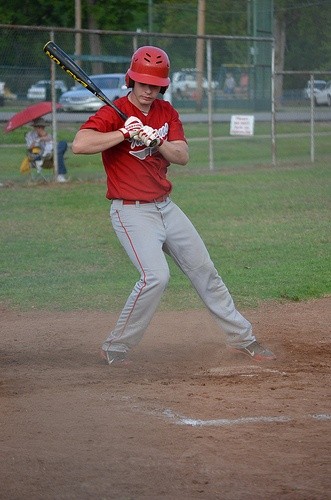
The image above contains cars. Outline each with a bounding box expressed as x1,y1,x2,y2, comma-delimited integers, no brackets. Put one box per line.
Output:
27,80,68,99
60,73,132,111
314,81,331,106
305,80,326,98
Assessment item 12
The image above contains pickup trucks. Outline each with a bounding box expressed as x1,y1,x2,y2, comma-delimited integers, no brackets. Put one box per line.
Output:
171,71,217,98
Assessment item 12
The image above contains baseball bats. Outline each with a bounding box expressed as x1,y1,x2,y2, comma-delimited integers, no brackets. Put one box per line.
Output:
42,40,158,148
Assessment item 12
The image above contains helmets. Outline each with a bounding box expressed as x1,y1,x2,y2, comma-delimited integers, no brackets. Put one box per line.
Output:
125,45,171,95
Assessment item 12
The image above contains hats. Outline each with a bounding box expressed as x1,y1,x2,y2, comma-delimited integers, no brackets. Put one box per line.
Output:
33,118,48,126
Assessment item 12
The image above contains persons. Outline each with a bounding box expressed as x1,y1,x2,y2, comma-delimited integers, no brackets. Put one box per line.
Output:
223,72,251,100
26,118,70,184
72,47,280,369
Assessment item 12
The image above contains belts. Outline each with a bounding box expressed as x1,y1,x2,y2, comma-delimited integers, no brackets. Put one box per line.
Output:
123,197,165,204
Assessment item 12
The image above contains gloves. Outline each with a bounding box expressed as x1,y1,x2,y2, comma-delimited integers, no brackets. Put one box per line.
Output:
119,116,144,140
134,125,163,147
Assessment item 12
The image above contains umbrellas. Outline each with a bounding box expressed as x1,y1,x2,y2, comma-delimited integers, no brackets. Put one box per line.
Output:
4,100,62,131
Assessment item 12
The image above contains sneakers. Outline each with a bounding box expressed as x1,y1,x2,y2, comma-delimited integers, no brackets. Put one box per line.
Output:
230,340,277,362
100,350,135,368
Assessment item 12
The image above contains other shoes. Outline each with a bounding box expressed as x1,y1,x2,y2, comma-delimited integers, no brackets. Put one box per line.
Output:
57,175,65,182
